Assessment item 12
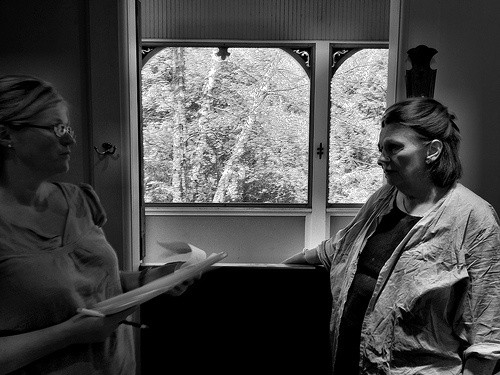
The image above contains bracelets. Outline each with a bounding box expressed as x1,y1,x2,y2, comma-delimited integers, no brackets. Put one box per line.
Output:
139,267,152,287
302,247,308,255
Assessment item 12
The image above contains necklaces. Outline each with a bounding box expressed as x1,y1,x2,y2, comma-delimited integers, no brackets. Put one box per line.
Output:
402,198,416,214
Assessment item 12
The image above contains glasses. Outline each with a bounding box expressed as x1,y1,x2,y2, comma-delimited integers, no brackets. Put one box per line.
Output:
19,119,74,136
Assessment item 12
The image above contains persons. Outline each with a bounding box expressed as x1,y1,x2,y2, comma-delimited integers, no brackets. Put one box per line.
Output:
0,75,200,375
279,96,500,375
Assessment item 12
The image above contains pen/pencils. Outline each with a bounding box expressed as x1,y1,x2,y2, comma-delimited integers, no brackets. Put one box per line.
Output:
76,306,147,331
